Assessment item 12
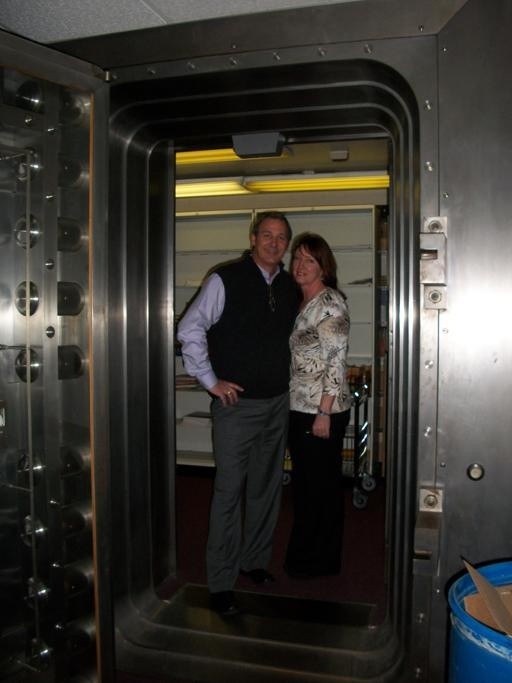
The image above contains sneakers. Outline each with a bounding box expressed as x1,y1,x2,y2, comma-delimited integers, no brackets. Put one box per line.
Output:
219,596,246,616
245,569,275,586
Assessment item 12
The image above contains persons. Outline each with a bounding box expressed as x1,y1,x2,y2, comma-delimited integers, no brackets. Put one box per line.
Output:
176,211,303,624
283,232,352,580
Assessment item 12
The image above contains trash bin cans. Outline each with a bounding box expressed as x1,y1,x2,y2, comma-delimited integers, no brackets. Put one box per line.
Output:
448,561,512,683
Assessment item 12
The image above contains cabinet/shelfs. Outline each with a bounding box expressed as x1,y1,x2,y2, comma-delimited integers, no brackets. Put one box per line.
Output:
171,206,380,478
283,383,378,510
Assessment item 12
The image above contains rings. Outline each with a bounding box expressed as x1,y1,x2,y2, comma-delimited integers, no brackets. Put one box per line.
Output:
228,390,231,395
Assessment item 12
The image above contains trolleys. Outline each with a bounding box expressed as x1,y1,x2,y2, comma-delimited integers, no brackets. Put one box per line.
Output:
284,365,376,508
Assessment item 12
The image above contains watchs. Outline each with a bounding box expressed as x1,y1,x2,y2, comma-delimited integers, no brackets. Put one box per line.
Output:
318,408,330,416
